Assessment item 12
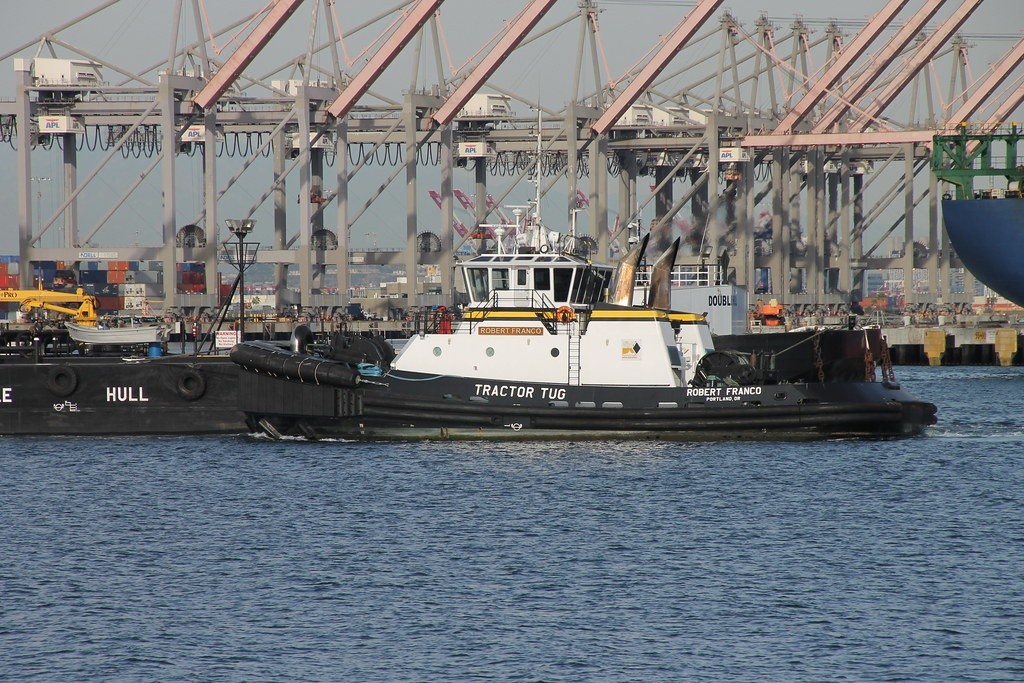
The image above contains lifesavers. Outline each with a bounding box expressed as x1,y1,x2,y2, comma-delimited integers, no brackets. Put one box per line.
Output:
46,365,78,398
176,367,207,402
556,305,575,322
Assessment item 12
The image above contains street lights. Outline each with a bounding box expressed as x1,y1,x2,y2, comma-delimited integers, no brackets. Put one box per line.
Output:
222,219,261,343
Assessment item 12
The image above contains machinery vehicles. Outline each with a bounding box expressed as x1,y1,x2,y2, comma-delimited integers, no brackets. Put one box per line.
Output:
0,284,101,330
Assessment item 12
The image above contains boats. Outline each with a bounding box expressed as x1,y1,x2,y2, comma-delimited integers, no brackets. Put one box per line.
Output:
64,321,173,346
231,98,940,448
0,334,251,435
